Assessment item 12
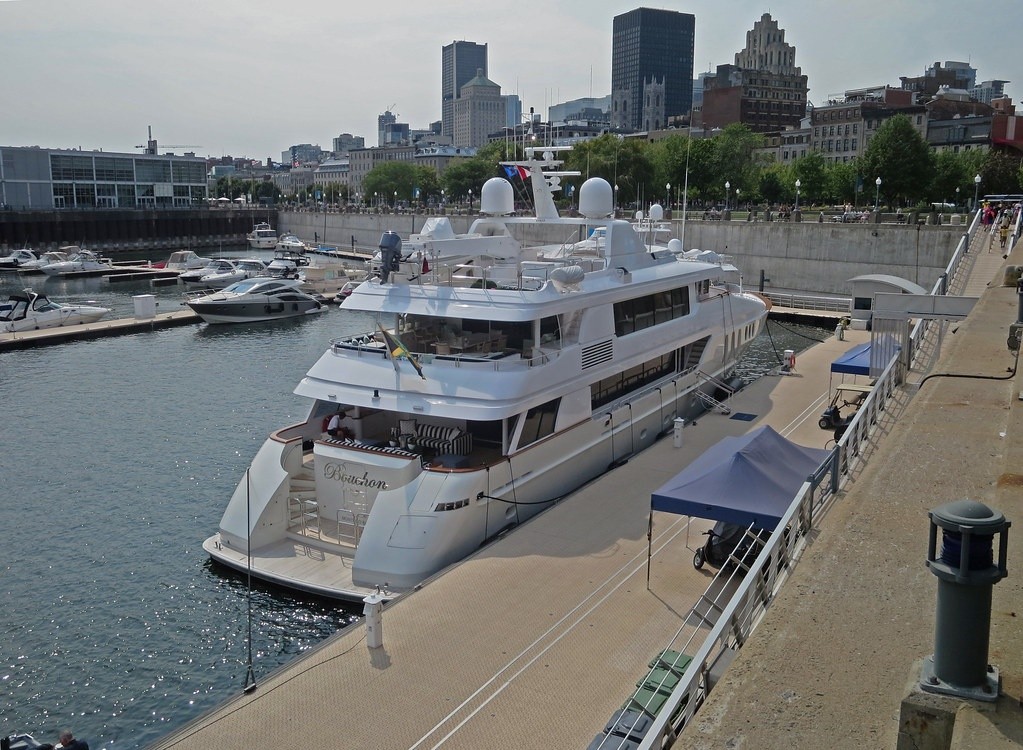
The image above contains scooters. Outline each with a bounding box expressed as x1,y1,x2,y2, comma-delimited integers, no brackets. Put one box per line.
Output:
690,526,771,578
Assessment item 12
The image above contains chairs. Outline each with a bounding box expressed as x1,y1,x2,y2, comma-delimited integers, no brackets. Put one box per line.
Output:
414,330,431,352
436,343,452,358
478,329,508,353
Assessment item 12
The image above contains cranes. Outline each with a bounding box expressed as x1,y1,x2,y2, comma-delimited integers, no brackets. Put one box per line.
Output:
135,144,203,154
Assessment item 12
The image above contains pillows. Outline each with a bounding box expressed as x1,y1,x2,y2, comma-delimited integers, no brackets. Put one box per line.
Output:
448,426,462,444
400,419,417,435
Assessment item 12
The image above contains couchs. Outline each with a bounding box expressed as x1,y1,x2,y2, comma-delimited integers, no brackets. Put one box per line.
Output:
322,438,432,470
390,424,472,455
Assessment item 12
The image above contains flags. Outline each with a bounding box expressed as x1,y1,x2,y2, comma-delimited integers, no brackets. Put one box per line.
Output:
378,324,426,381
504,165,530,180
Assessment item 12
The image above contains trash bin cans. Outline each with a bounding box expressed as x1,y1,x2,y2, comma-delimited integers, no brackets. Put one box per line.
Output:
603,709,654,745
586,732,640,750
636,666,679,697
622,688,669,721
647,648,694,677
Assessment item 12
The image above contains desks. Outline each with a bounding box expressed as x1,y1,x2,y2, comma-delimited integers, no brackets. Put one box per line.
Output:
431,332,498,354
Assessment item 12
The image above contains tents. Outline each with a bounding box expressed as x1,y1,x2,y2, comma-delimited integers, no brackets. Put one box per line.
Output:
650,424,831,589
827,334,901,409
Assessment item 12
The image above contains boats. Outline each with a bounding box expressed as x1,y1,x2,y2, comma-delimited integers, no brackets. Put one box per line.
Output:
198,175,772,611
182,276,328,326
0,244,368,304
0,287,112,335
275,232,305,255
246,222,277,250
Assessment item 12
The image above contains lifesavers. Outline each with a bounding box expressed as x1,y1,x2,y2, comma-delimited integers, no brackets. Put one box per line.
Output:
790,355,796,366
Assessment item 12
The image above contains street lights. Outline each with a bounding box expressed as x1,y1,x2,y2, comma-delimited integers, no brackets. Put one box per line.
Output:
441,189,444,214
355,192,358,214
724,180,731,211
666,183,671,210
873,177,881,212
955,187,960,213
571,185,576,210
970,174,981,212
735,189,740,211
374,192,377,214
793,178,801,211
339,193,342,213
613,184,618,210
394,191,397,214
468,189,472,210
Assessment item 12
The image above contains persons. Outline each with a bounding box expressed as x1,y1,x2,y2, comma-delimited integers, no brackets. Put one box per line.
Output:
327,412,350,441
670,199,1023,248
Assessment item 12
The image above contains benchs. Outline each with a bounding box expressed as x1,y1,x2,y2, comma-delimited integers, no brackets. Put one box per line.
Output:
843,213,870,223
